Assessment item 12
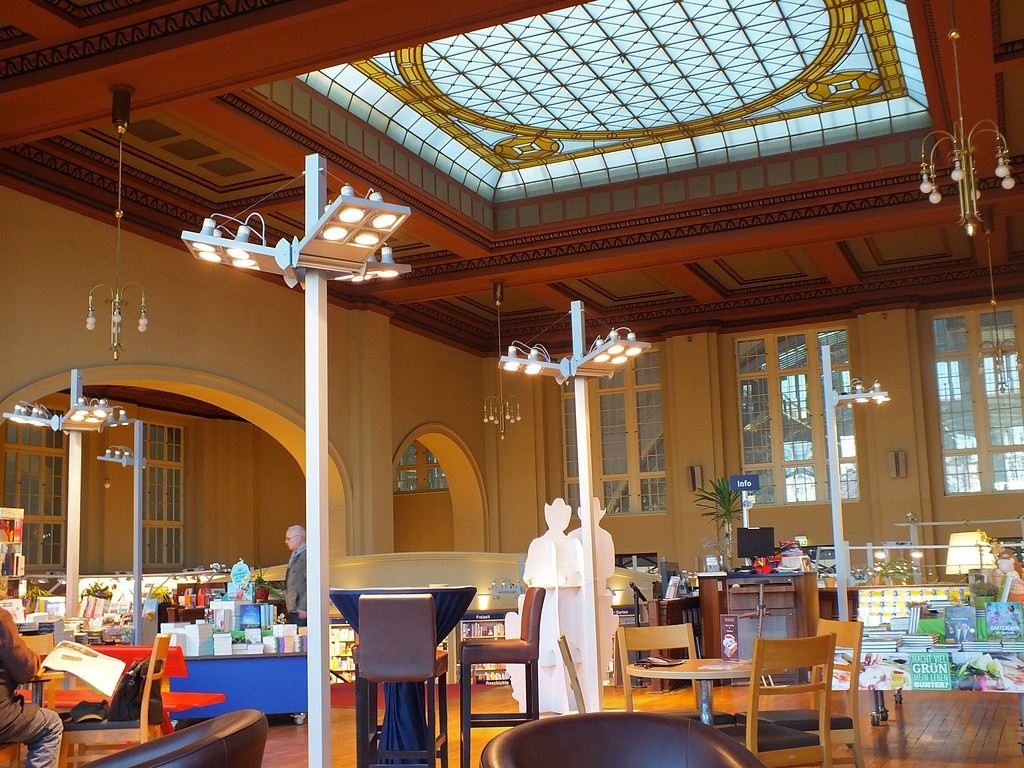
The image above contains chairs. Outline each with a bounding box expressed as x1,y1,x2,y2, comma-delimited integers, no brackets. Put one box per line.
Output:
734,619,865,768
557,634,586,714
716,631,837,768
58,633,173,768
617,623,737,728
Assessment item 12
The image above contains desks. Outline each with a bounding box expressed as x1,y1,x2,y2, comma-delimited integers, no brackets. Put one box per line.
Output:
29,668,72,711
169,652,309,731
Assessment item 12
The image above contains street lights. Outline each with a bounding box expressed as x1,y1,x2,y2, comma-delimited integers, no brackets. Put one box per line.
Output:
1,369,137,615
822,344,890,621
96,419,143,643
499,301,651,714
179,154,413,768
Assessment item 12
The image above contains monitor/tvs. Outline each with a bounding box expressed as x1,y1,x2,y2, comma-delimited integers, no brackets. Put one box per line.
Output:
737,527,775,560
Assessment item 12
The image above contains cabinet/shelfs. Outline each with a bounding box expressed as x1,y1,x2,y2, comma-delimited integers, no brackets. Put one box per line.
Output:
460,620,511,685
697,571,820,688
338,650,339,651
328,624,355,684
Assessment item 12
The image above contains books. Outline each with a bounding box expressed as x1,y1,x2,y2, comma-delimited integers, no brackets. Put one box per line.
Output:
636,655,685,667
425,637,450,684
80,595,160,645
39,640,126,697
659,562,701,663
603,606,640,687
462,622,511,685
779,539,803,557
330,629,356,682
696,553,727,577
829,590,1024,650
0,516,87,648
160,598,307,656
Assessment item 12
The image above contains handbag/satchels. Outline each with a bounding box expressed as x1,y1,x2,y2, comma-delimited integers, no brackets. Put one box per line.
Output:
108,658,165,724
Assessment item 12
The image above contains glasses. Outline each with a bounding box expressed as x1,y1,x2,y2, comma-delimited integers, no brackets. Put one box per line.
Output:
286,534,301,541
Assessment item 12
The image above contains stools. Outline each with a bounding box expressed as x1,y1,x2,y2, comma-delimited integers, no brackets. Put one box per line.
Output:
459,587,546,768
78,710,268,768
480,711,768,768
353,593,449,768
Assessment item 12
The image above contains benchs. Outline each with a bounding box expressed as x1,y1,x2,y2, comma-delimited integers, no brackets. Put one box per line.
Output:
14,645,226,736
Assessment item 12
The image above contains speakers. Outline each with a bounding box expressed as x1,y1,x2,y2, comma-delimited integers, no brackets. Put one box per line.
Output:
686,465,702,492
888,450,907,478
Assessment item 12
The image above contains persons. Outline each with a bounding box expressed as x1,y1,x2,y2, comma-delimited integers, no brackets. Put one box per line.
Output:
0,607,64,768
283,525,306,627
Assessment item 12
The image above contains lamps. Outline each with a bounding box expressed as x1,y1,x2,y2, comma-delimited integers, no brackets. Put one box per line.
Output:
97,445,134,467
292,183,411,277
919,0,1015,236
328,242,412,282
483,278,521,440
181,212,298,290
570,327,651,379
107,404,136,427
86,84,148,360
945,531,999,607
838,377,891,404
498,340,570,386
977,205,1024,393
3,400,59,432
62,395,113,434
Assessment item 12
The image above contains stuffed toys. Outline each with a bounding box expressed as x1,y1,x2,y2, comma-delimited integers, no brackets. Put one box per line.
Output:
996,551,1024,603
